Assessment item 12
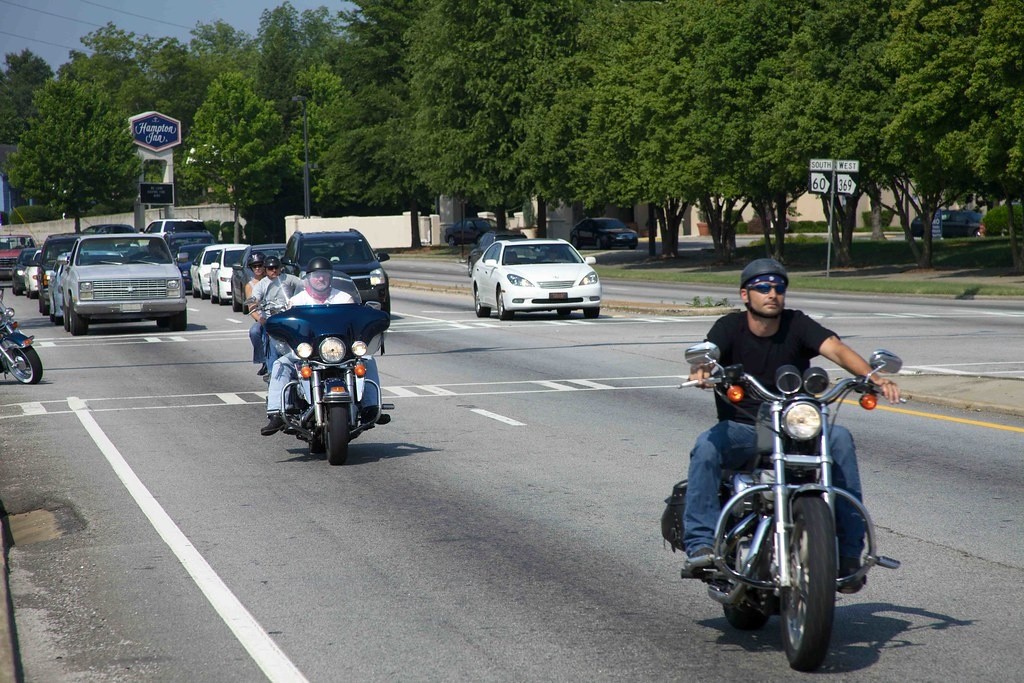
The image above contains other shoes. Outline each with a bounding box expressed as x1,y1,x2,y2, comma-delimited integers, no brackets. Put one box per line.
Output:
685,547,716,572
838,558,864,593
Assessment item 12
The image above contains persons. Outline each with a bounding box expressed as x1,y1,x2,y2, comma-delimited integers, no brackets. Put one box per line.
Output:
14,237,30,249
130,239,168,261
540,247,571,262
336,243,357,262
681,258,900,595
260,257,391,436
245,251,310,375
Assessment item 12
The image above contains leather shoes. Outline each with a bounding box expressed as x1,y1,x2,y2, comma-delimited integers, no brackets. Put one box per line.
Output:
260,413,285,436
257,362,267,375
360,406,390,425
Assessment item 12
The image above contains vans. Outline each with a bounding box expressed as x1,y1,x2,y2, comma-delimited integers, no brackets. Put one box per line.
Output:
0,233,36,279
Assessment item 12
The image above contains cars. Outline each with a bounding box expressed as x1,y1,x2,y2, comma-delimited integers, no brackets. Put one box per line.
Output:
45,252,71,325
173,243,289,315
570,218,638,250
467,232,528,277
82,224,136,234
445,218,497,247
9,246,43,299
911,205,985,238
471,237,602,321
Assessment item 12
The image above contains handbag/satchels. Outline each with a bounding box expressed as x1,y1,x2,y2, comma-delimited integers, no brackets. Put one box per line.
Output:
660,478,735,552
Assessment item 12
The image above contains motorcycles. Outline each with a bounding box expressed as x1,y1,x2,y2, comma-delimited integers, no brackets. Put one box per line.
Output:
0,288,43,385
676,341,910,673
243,268,395,466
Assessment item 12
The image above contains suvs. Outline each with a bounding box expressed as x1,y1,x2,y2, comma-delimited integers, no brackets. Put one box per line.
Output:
139,219,207,235
166,230,216,262
280,228,390,318
57,233,190,336
29,233,89,315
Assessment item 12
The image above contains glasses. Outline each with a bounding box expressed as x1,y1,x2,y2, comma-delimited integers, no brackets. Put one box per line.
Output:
743,284,786,293
251,264,262,268
267,267,279,270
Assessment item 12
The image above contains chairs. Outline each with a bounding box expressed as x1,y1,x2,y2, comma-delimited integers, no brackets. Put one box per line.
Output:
0,242,11,249
503,251,518,265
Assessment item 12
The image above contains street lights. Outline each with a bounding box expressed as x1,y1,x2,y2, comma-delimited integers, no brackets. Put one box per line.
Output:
292,96,310,219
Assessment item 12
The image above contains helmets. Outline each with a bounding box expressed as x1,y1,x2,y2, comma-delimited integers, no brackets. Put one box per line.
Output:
281,256,295,266
263,255,281,267
247,251,266,268
308,256,332,271
740,258,788,289
